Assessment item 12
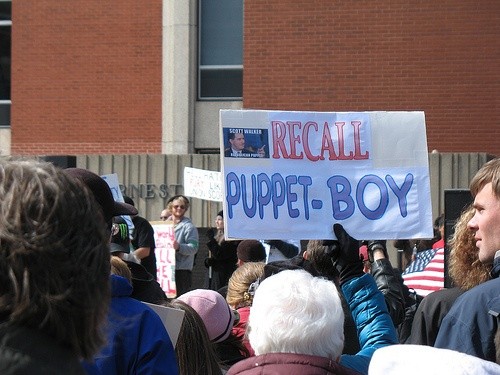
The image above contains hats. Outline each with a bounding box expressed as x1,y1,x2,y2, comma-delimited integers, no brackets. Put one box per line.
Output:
259,256,318,278
109,217,131,254
236,239,267,263
177,288,235,344
64,167,138,223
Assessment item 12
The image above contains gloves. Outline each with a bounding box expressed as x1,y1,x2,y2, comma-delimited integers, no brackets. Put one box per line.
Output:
206,225,215,239
362,240,386,253
321,223,365,286
206,256,214,266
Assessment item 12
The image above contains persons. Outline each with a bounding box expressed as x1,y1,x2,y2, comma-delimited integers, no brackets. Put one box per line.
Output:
225,130,266,158
0,141,500,375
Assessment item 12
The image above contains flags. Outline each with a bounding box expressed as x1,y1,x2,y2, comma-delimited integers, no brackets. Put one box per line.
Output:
401,248,444,298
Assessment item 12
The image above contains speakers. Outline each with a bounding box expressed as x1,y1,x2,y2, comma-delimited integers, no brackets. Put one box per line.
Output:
444,188,476,288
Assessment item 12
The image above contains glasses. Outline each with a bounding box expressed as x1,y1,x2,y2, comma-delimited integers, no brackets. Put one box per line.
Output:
160,216,168,219
171,204,188,209
